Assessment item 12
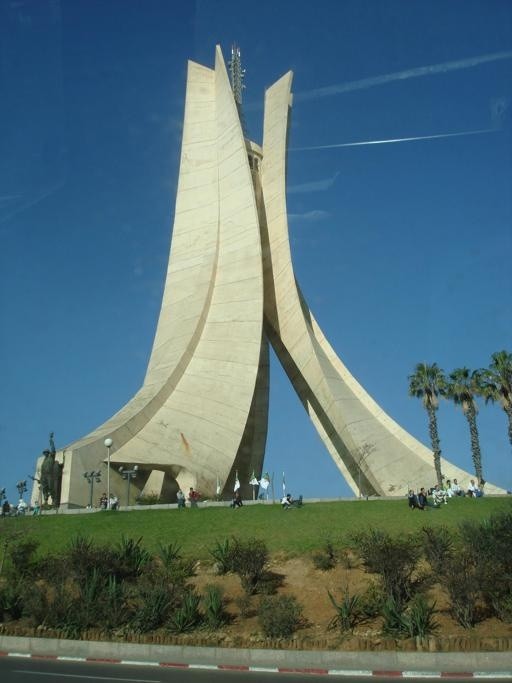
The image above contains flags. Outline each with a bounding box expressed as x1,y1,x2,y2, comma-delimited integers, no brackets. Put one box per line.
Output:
249,471,259,485
282,472,286,490
259,472,269,490
233,472,240,493
216,473,221,494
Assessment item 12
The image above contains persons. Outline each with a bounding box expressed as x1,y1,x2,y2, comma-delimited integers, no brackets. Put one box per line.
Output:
3,500,10,517
408,479,482,510
188,487,200,509
233,492,241,509
41,432,57,507
176,489,186,509
281,494,293,510
110,493,119,510
32,501,40,516
99,493,108,510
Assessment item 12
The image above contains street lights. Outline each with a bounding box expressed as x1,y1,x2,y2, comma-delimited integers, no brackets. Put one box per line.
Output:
104,437,113,510
118,464,139,506
0,487,7,507
16,479,28,499
83,469,101,506
